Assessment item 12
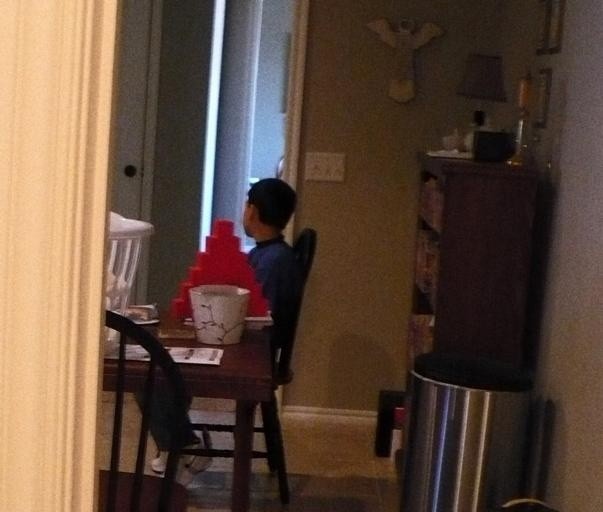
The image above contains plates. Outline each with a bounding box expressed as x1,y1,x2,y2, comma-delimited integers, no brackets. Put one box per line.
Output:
116,317,160,326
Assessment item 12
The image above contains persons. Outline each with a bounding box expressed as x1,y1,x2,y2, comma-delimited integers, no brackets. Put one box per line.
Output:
132,177,299,487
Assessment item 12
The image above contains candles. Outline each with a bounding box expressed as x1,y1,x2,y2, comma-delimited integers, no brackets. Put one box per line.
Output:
516,78,530,110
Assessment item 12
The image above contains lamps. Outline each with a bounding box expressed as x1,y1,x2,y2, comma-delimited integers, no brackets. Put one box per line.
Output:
454,54,508,158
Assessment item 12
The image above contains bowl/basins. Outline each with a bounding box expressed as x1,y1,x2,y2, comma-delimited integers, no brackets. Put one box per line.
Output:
473,130,516,164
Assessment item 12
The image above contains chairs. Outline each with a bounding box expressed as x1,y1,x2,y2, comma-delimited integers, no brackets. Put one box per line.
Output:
104,309,198,512
163,228,318,509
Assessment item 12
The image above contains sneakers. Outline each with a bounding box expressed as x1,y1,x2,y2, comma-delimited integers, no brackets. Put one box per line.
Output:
151,449,170,474
174,440,214,487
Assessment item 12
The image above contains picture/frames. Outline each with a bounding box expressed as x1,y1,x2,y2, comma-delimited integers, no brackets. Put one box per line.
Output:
545,2,564,51
532,67,552,128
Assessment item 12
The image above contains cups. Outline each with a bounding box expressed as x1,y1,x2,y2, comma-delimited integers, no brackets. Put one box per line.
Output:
442,135,463,150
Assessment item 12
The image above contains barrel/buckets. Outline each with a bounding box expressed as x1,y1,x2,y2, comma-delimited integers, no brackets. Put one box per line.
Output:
188,284,252,346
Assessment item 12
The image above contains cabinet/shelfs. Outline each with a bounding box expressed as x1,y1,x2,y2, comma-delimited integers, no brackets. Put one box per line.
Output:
406,155,540,371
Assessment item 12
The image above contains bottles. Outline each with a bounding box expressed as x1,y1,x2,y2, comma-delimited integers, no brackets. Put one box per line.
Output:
464,112,487,159
506,74,534,166
520,68,555,182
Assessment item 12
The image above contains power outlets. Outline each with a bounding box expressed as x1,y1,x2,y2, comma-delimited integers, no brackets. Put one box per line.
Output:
306,151,346,182
